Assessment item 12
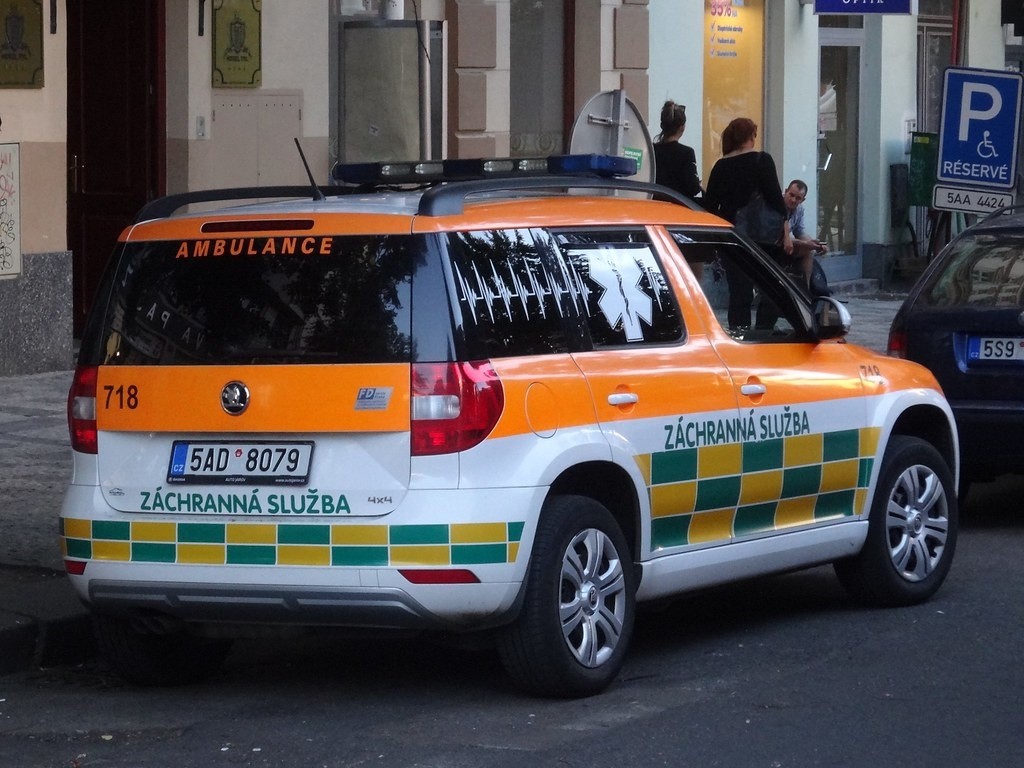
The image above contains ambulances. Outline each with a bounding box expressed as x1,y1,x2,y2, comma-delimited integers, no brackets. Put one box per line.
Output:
62,152,960,704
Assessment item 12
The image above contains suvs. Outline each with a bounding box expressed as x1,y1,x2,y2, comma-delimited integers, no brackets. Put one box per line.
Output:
887,203,1023,426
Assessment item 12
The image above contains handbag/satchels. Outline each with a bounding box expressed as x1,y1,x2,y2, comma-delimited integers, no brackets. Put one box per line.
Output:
810,259,833,297
736,151,784,247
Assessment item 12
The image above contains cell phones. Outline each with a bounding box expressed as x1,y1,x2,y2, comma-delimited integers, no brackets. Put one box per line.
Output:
817,241,827,246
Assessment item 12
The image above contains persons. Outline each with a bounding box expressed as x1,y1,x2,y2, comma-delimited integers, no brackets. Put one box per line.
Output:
649,100,701,280
704,117,787,330
784,179,828,290
